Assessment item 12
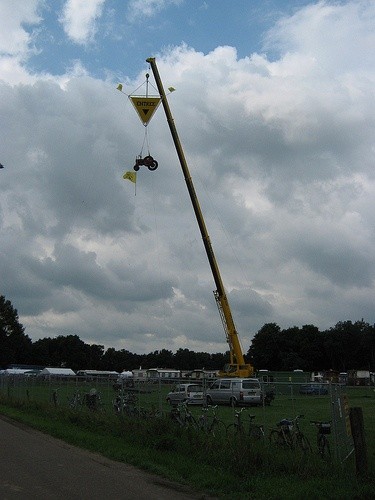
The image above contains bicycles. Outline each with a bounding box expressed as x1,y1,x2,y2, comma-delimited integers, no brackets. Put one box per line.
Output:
70,390,332,467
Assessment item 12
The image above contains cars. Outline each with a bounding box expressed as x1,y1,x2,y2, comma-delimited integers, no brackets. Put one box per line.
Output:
167,384,205,406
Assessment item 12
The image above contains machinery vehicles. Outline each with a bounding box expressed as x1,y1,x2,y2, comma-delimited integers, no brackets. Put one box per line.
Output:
147,60,255,381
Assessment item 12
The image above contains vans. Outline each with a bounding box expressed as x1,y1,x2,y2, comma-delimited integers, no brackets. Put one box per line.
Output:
204,377,263,407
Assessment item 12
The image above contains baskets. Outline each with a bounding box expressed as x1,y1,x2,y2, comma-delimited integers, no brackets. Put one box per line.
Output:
320,426,331,435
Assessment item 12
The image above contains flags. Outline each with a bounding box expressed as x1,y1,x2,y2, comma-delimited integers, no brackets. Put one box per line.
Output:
123,171,136,183
116,83,122,91
168,86,175,93
